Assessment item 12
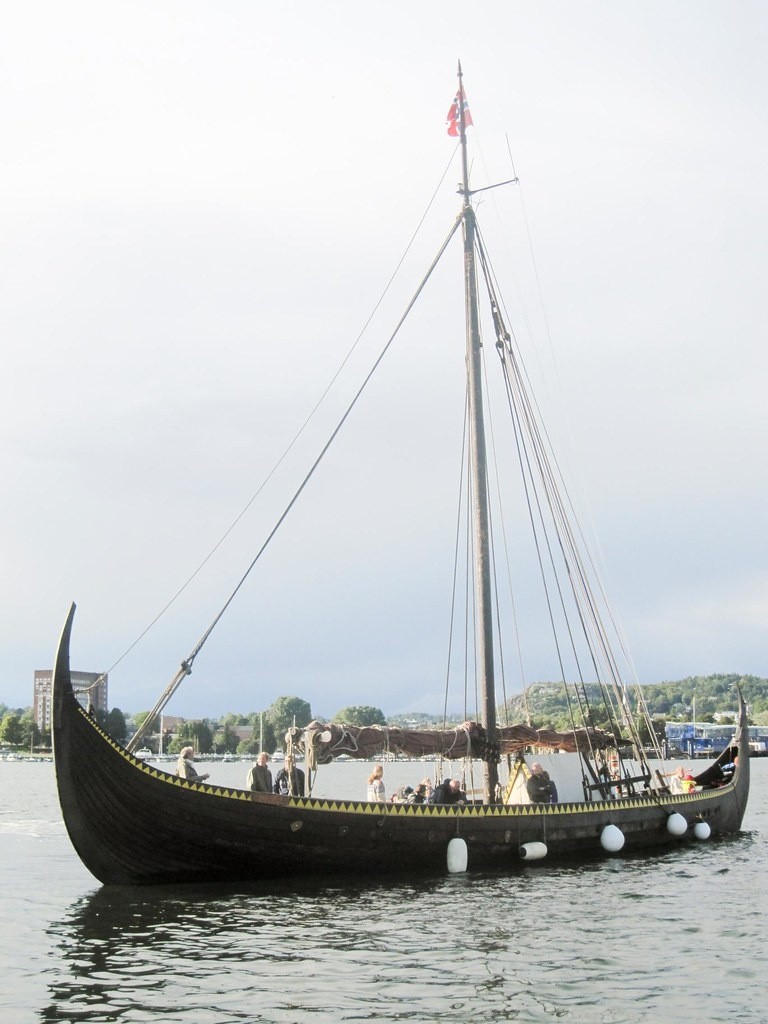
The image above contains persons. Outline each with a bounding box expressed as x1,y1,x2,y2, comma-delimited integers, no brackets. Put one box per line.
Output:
247,752,272,793
392,778,435,804
367,765,386,803
176,746,210,783
722,755,739,783
669,766,695,795
430,778,467,805
273,756,304,796
528,764,558,803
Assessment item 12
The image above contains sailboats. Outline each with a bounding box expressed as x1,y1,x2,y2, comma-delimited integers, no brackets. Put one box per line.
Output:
48,58,756,890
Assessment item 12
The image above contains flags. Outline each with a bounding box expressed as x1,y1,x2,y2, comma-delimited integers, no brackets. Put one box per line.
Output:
447,87,473,137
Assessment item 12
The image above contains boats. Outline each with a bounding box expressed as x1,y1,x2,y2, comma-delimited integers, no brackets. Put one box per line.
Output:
0,732,53,762
241,756,252,763
272,757,279,763
222,755,234,762
